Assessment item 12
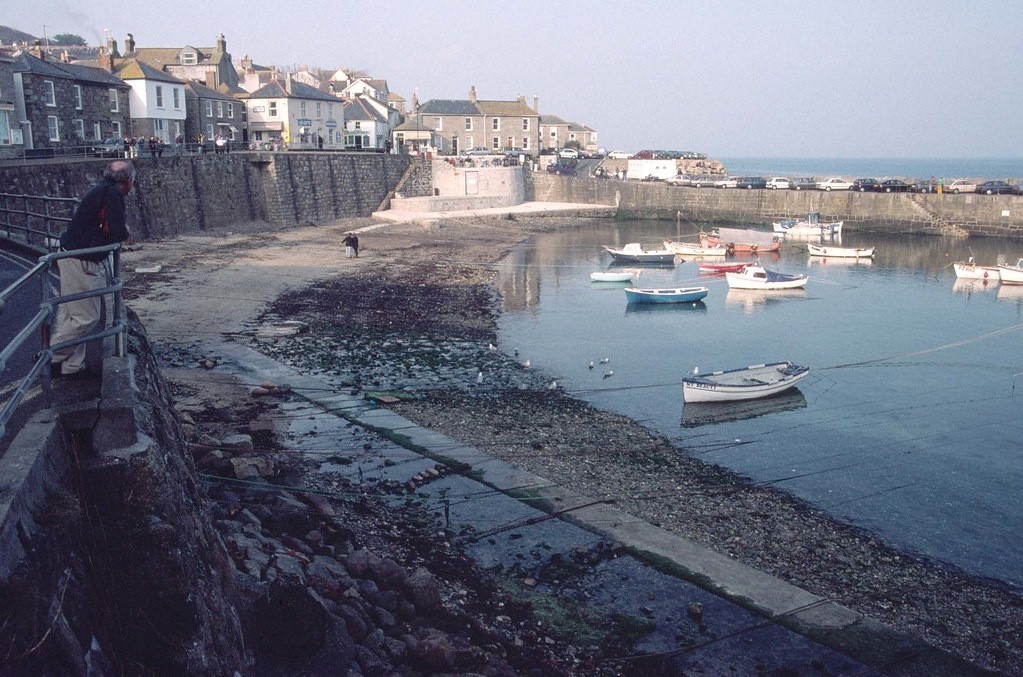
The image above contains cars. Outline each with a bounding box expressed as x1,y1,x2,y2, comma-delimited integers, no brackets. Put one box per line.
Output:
975,180,1013,195
714,176,738,188
690,174,722,188
816,178,854,191
465,143,708,159
665,174,696,187
1012,182,1023,194
909,180,938,193
942,180,977,193
872,179,914,193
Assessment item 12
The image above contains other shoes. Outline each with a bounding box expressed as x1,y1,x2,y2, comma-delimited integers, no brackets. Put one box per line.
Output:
32,352,61,379
62,367,101,380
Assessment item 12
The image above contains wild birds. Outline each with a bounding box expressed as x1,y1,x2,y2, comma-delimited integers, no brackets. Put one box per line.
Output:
588,361,594,370
601,370,614,379
548,382,557,390
477,371,483,383
489,343,498,353
513,348,520,360
598,357,609,364
515,359,531,372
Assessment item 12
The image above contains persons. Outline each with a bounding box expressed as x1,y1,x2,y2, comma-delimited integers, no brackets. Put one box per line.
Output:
212,131,232,154
197,133,208,155
445,155,508,167
318,135,324,148
123,135,130,159
46,160,136,380
157,136,165,157
175,134,184,157
340,233,358,258
149,136,157,158
600,165,626,180
129,135,145,160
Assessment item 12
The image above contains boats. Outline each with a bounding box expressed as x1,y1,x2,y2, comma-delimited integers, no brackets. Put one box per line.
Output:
589,216,1023,289
624,287,708,304
679,386,809,428
680,359,810,401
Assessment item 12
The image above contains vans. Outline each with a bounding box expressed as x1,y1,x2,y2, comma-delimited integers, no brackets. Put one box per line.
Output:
736,176,766,189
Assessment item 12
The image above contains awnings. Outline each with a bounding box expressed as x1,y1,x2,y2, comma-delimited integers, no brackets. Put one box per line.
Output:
250,122,283,131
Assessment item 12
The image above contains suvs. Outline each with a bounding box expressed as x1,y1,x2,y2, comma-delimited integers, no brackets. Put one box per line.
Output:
789,178,817,190
546,163,578,176
765,177,793,189
852,178,882,192
91,137,126,157
